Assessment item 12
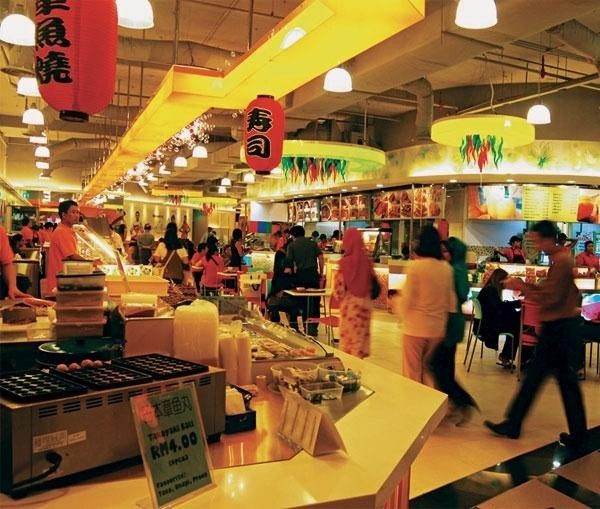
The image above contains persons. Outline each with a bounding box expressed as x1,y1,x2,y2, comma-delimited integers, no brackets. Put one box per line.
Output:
45,199,103,300
403,226,457,387
494,235,530,264
574,240,600,270
577,320,600,379
337,227,376,361
481,220,590,450
118,211,246,297
481,268,519,370
14,214,59,260
272,225,344,334
432,235,478,425
1,223,29,301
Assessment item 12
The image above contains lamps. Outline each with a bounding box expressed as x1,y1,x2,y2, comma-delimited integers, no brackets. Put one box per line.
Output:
115,0,155,29
324,63,357,96
527,72,552,128
453,0,501,32
99,110,285,194
0,1,51,204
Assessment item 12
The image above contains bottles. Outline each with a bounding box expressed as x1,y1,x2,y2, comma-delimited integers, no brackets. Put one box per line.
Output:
226,262,255,275
256,375,265,392
577,194,595,222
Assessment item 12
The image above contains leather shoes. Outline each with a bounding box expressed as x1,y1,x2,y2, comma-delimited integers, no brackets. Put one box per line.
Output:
558,433,591,453
483,417,522,441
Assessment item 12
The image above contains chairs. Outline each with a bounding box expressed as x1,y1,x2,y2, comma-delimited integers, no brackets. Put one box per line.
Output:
463,296,515,374
189,263,341,348
582,291,600,376
517,301,549,379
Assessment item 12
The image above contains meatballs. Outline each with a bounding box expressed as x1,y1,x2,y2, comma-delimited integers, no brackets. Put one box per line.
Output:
57,359,104,373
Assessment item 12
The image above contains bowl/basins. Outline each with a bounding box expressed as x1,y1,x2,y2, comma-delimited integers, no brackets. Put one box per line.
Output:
271,362,363,392
321,199,350,221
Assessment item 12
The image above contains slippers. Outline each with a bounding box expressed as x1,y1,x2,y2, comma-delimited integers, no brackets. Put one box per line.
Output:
495,357,516,371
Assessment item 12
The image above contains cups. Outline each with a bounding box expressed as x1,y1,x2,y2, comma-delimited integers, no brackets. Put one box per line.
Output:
219,332,251,384
486,196,518,219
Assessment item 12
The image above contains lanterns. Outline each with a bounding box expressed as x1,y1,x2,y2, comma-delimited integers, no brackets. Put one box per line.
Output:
242,95,286,173
34,0,118,123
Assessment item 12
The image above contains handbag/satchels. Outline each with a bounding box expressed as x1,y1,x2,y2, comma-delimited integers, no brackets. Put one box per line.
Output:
369,274,382,302
152,267,166,279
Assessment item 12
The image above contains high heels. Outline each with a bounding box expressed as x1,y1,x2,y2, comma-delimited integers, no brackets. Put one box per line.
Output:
455,395,481,429
